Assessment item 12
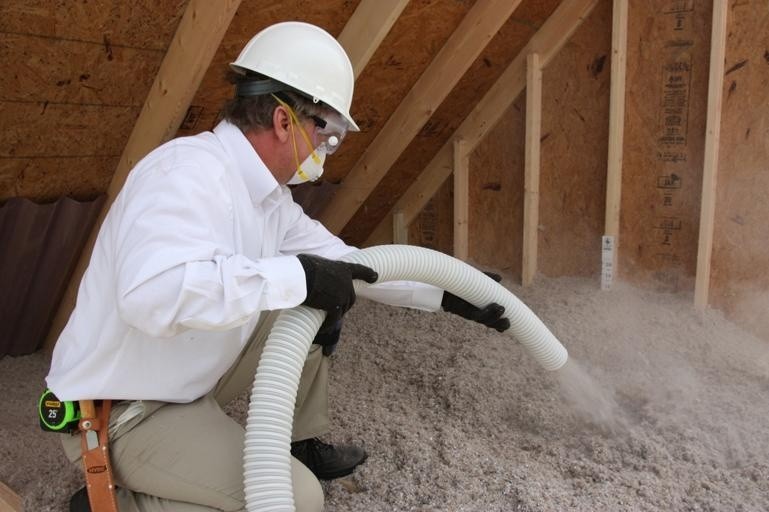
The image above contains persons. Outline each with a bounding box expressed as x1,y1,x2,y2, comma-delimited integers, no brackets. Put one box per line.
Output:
38,20,511,510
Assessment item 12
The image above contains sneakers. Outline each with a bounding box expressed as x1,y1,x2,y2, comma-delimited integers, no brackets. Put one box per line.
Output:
290,437,367,479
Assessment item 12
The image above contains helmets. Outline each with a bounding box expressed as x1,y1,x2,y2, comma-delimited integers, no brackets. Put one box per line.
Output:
228,21,361,132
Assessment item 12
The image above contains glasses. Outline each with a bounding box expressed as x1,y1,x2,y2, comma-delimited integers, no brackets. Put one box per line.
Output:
317,115,347,155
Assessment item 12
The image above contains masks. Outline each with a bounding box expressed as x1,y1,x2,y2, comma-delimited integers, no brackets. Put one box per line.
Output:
291,116,326,186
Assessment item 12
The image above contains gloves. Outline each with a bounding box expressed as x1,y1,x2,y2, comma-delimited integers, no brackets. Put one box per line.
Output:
295,254,378,355
442,271,510,333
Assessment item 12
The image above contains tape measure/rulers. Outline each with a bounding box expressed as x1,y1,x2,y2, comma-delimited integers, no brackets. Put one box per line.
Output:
38,387,81,434
